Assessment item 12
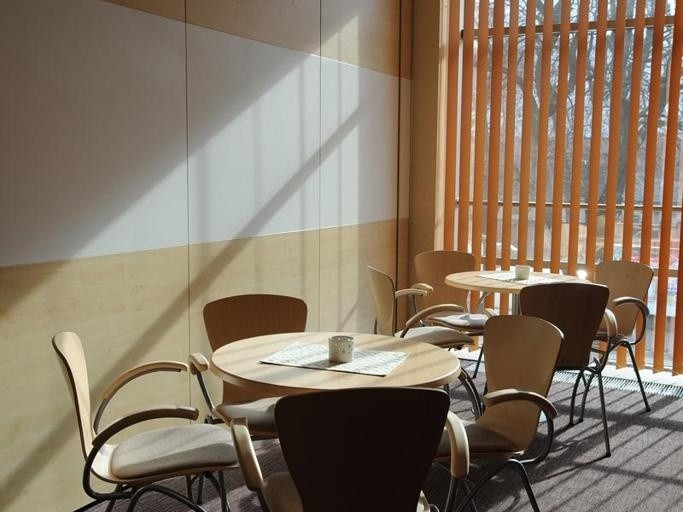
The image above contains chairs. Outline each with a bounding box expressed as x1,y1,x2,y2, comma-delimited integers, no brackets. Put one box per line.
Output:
367,265,481,420
482,283,611,457
409,250,499,378
203,294,309,490
54,331,239,511
229,390,468,512
581,260,655,422
433,315,564,512
